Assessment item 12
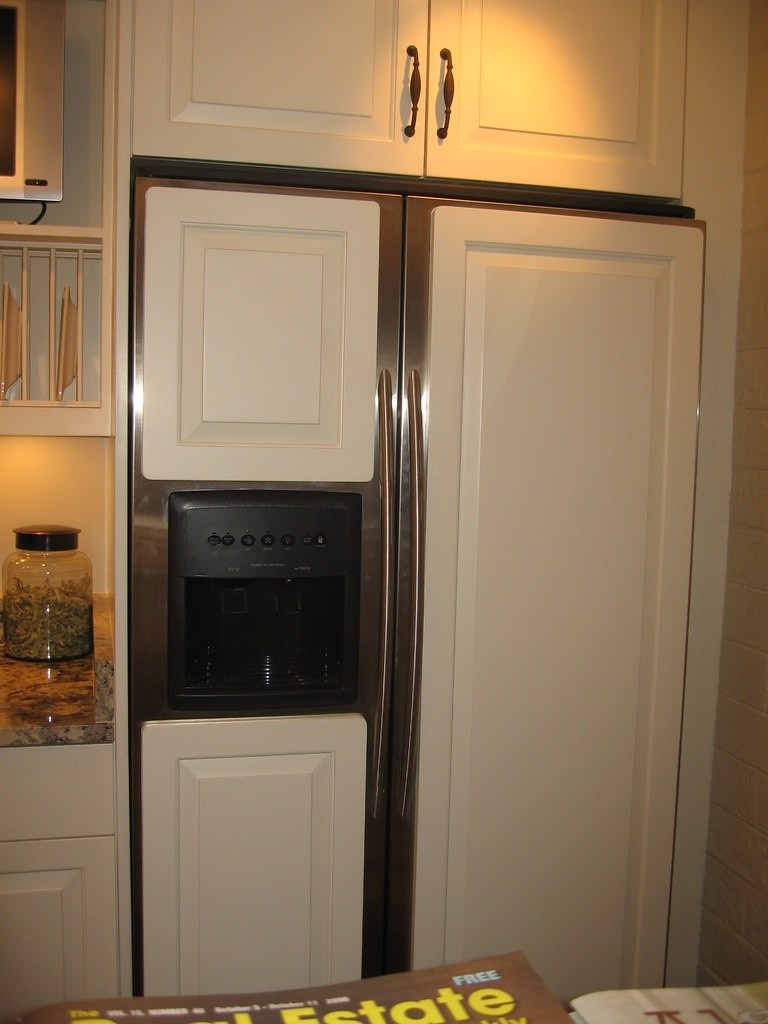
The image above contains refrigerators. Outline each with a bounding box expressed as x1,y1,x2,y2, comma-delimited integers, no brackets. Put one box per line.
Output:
129,178,706,996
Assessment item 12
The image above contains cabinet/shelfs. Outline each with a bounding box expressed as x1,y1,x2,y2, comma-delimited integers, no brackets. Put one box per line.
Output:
115,2,699,187
139,712,366,998
411,204,707,986
0,742,129,1022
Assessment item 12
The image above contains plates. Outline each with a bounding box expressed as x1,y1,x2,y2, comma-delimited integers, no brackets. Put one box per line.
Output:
57,286,78,403
0,282,21,400
